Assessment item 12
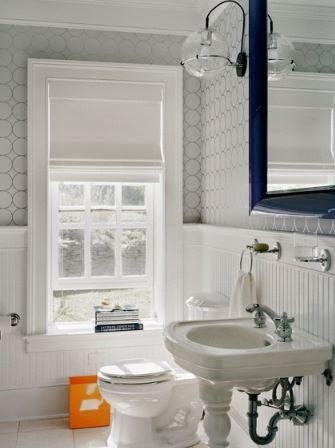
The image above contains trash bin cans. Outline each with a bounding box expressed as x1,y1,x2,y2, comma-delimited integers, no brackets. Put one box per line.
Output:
69,375,110,430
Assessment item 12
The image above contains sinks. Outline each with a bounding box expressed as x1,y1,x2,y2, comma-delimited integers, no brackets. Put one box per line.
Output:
163,317,332,395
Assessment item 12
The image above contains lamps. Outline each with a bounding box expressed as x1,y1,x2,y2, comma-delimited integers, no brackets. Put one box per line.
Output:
181,26,234,78
267,28,297,81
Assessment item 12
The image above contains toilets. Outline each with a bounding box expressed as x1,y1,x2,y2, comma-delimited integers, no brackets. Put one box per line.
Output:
96,292,230,448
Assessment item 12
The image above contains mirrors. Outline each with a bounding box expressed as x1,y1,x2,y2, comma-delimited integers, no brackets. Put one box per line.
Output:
249,0,335,220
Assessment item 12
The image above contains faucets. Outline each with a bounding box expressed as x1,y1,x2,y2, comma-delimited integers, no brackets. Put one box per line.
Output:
245,303,281,330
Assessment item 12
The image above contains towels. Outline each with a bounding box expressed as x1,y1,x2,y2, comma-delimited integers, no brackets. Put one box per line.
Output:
228,271,257,318
